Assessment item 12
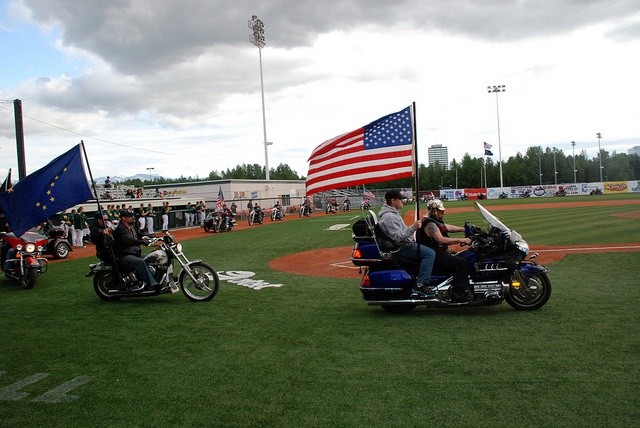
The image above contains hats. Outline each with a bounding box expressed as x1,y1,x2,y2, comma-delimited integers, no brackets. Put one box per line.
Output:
384,189,407,199
120,210,135,217
427,199,446,211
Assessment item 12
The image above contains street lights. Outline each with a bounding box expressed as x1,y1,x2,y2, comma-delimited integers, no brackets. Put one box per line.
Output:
596,133,604,182
571,141,577,183
537,153,543,185
248,15,270,180
552,146,557,185
487,85,506,187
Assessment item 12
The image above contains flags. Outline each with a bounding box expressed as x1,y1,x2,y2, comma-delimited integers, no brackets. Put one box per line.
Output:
304,103,413,197
0,141,97,238
215,189,225,211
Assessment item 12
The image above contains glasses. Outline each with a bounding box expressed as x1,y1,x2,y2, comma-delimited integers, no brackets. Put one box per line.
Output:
103,219,110,221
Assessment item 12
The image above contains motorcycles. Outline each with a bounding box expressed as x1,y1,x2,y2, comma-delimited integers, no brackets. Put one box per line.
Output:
326,203,340,214
351,202,551,314
521,193,530,197
477,195,484,200
42,229,72,259
299,204,313,217
85,228,218,302
203,210,236,233
499,194,508,199
270,205,285,221
248,208,265,226
590,190,603,195
0,223,49,289
555,192,566,196
361,200,371,210
343,202,351,212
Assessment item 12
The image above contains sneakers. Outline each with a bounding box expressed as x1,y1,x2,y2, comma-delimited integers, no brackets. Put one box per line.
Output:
413,282,432,294
451,288,473,301
153,282,165,289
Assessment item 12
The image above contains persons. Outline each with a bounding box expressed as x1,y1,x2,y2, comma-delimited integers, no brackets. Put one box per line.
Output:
162,200,169,231
302,200,310,217
92,209,118,290
147,203,155,233
138,202,148,229
122,203,127,209
59,205,90,246
344,197,351,212
183,200,207,226
103,176,142,198
105,204,113,227
113,210,162,289
253,202,263,223
96,204,105,214
223,203,232,224
117,204,121,219
417,198,471,303
274,200,282,216
112,204,116,223
378,190,437,297
128,206,134,211
